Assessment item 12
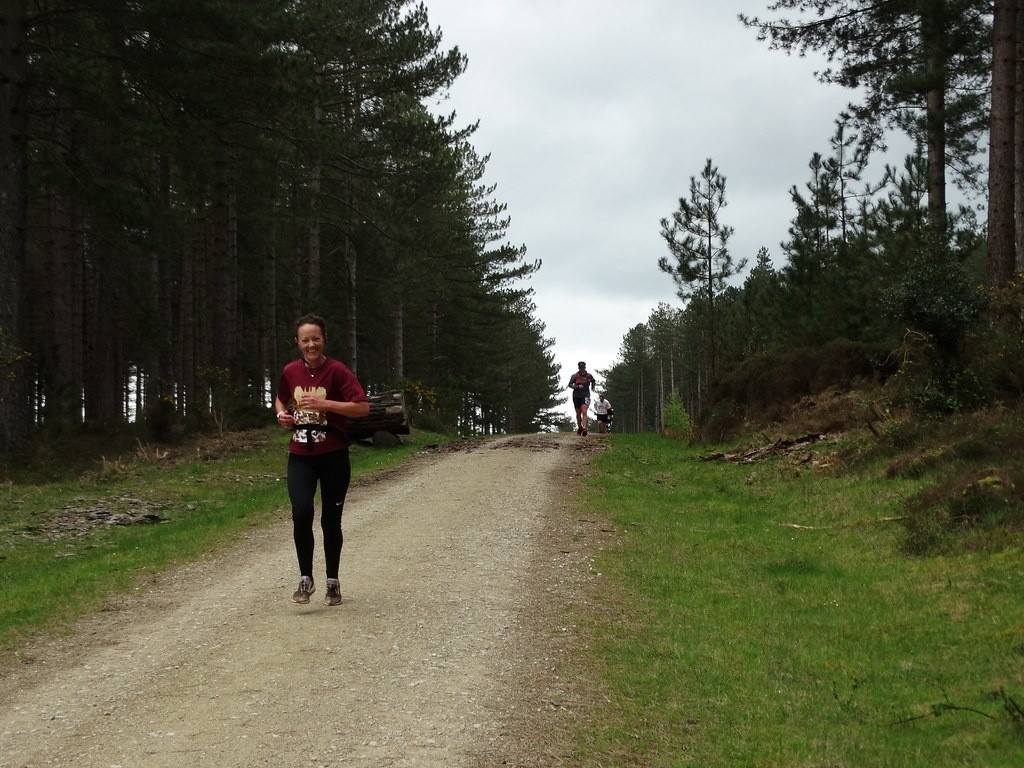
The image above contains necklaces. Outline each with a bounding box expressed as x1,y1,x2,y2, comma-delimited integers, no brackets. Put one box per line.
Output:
303,358,322,378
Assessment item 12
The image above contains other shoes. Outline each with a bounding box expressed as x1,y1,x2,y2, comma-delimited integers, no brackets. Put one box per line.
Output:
582,427,587,436
578,426,582,435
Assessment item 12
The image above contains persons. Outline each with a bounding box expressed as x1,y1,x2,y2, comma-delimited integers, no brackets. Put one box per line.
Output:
275,313,370,606
568,362,596,436
594,394,612,433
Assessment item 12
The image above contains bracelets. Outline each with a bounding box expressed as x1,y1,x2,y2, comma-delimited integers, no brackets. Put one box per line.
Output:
277,411,285,423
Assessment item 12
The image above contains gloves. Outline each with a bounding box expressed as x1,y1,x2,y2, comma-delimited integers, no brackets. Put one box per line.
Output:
579,385,584,390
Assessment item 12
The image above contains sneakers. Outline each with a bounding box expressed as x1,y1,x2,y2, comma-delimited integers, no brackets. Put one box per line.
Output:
291,576,315,604
324,578,342,605
